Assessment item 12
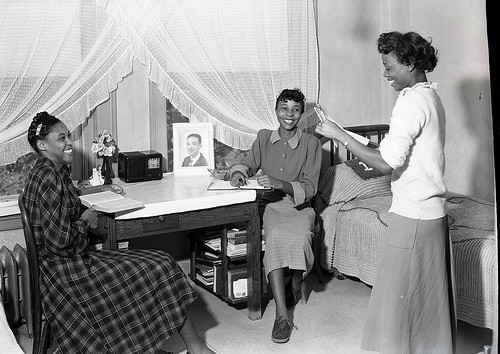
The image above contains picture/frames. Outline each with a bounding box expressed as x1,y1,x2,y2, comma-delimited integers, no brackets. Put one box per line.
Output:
173,122,216,176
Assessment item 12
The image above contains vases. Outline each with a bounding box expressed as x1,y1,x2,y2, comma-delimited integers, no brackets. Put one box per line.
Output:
100,156,114,185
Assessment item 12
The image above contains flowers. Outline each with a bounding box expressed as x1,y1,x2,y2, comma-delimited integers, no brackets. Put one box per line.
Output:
89,127,118,158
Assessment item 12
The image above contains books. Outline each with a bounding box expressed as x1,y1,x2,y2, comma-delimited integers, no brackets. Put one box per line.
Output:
77,191,144,213
196,226,265,298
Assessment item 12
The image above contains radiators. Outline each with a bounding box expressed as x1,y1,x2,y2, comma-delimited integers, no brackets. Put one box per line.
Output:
0,242,34,340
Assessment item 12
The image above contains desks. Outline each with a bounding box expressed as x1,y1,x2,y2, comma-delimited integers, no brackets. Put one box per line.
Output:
79,171,264,322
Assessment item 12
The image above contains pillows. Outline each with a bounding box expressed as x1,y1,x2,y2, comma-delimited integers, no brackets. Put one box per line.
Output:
322,157,393,200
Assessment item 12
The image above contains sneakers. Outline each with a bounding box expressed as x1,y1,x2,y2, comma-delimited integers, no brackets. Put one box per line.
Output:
270,315,297,343
285,290,300,311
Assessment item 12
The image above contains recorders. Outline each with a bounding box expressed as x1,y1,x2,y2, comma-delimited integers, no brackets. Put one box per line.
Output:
118,150,163,183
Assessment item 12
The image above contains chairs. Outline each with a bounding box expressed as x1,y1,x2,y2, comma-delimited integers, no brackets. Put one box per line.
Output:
17,189,50,354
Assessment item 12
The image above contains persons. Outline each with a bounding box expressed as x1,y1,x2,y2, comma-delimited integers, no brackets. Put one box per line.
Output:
230,89,323,343
182,133,208,167
21,111,217,354
314,30,458,354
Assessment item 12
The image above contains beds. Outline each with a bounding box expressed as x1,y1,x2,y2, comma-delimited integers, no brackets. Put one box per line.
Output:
316,123,497,328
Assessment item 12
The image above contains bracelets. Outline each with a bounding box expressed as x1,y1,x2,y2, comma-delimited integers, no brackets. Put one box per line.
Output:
345,137,352,149
